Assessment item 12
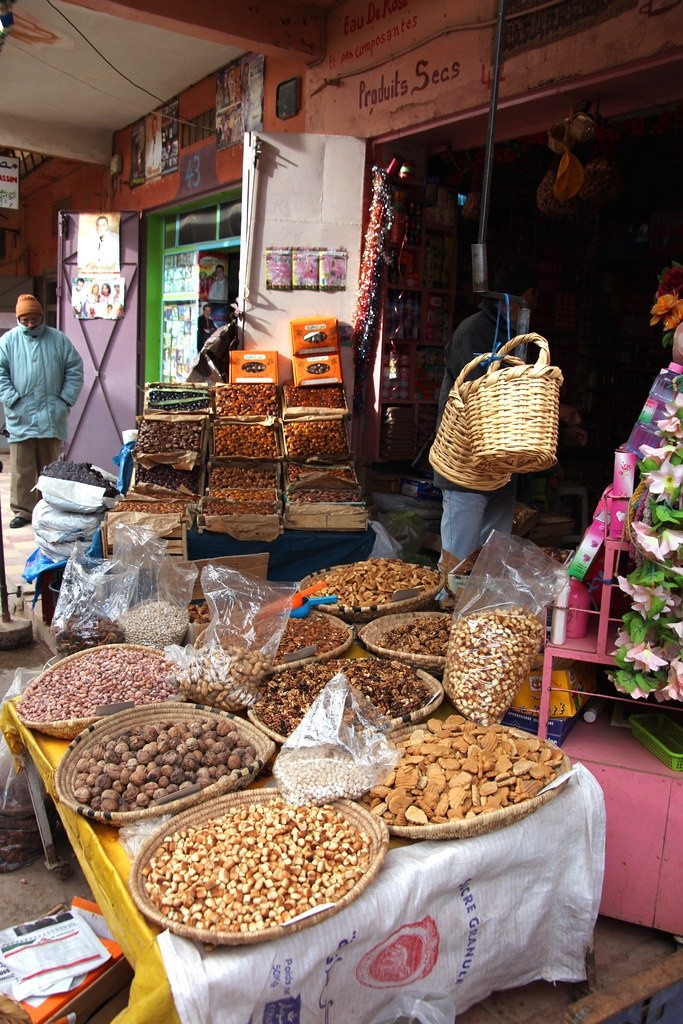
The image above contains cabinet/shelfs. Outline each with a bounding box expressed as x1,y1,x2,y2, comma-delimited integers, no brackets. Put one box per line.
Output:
601,220,672,448
539,362,683,946
500,184,599,349
360,177,458,500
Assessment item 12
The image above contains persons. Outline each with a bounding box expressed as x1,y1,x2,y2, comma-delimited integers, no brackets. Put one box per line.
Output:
0,293,85,528
72,277,123,318
95,215,119,267
147,116,161,175
161,126,179,170
240,63,250,131
209,264,228,300
432,267,582,601
222,72,229,105
133,126,145,178
197,304,217,353
216,109,240,147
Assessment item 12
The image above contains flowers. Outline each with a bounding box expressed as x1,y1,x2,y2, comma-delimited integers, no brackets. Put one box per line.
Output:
605,373,683,702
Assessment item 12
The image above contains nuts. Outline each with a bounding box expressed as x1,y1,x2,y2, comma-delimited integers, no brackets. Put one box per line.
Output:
72,716,256,813
174,544,570,743
16,647,180,723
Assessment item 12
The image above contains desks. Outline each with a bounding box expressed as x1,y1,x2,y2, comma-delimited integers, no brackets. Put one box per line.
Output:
108,522,376,582
0,694,606,1024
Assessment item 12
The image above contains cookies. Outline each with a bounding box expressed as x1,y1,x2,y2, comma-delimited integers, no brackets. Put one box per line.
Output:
361,714,566,827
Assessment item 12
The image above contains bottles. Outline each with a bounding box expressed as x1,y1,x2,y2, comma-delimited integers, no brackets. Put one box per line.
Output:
583,696,605,723
566,575,591,639
626,362,683,459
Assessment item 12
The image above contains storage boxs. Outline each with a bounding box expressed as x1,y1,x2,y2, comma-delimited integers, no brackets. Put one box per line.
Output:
366,235,455,499
0,895,135,1024
107,382,369,541
423,500,576,553
511,653,599,719
444,187,458,226
425,183,449,224
291,351,344,388
500,706,578,748
289,315,341,356
381,140,429,180
229,350,278,384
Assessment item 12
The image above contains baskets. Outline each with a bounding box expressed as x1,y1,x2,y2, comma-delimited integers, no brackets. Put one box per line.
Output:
54,702,276,826
17,644,187,740
298,557,447,623
428,352,526,491
128,785,389,944
193,606,354,679
357,611,453,676
345,724,571,840
459,333,564,473
247,658,444,747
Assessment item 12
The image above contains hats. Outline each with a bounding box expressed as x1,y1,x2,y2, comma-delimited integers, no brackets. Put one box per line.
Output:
15,295,43,318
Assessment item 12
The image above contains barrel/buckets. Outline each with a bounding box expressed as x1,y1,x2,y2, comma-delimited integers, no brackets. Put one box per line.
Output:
48,580,60,607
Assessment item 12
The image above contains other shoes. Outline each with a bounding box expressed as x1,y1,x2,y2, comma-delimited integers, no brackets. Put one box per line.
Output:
10,517,31,528
